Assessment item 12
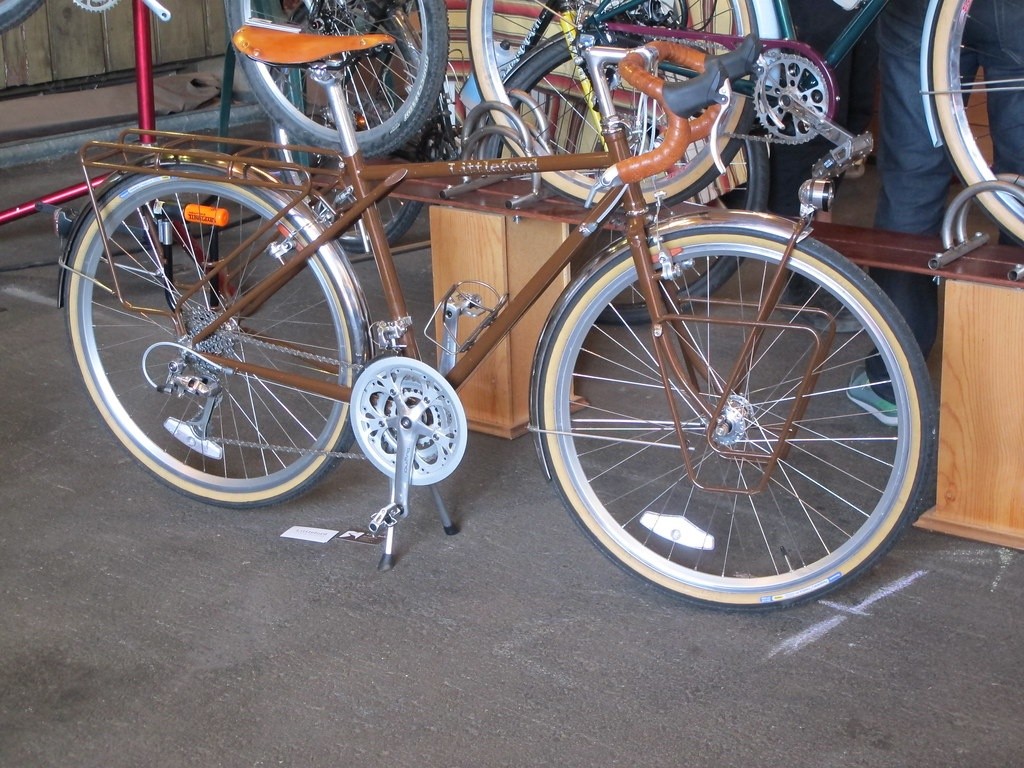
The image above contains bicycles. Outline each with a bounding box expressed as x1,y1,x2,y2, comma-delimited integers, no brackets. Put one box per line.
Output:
0,0,1022,327
51,26,938,615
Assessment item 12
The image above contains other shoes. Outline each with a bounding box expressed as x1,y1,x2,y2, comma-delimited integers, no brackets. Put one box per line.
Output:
845,364,899,426
780,288,862,333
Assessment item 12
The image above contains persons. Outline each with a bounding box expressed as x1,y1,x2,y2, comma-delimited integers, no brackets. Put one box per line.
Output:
761,0,879,332
845,0,1024,426
